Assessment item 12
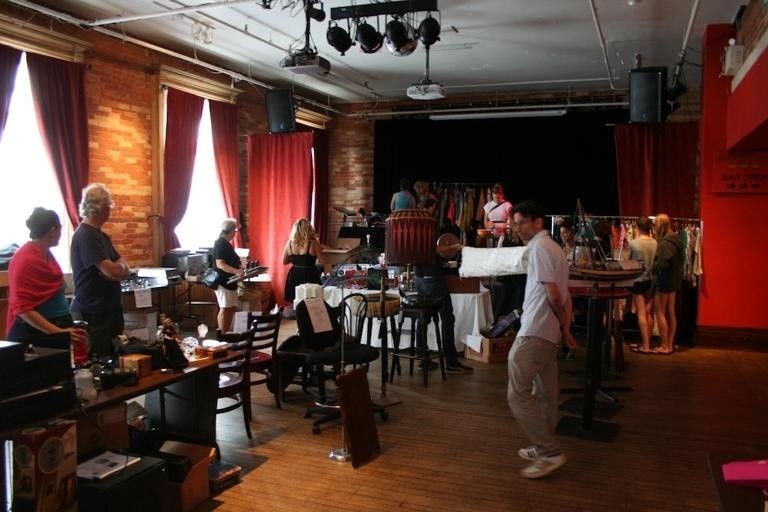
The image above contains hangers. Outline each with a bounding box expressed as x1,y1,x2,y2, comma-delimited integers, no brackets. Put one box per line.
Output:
431,180,489,195
557,214,698,232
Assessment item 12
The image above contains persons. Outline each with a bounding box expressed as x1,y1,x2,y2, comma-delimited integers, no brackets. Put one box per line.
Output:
644,212,685,355
555,213,628,265
482,184,514,246
411,193,475,375
282,217,332,304
69,183,131,355
210,218,246,331
412,180,439,215
501,201,579,480
4,206,88,366
389,177,416,210
627,216,657,354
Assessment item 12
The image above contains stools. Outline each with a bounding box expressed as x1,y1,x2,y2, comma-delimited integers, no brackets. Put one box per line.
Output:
389,296,449,387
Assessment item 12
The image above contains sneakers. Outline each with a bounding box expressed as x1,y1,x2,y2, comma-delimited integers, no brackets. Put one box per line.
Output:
445,362,474,374
417,360,440,370
519,450,566,480
518,445,541,463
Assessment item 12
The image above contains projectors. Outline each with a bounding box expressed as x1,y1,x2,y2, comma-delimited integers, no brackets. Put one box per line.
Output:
406,78,446,101
279,51,330,76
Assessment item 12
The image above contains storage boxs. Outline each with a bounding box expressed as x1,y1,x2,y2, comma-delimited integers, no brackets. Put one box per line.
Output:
320,235,361,263
10,416,78,511
77,399,131,453
463,329,518,364
147,441,217,512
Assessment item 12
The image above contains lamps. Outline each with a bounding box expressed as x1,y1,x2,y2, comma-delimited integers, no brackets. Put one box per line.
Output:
381,206,439,290
327,0,440,56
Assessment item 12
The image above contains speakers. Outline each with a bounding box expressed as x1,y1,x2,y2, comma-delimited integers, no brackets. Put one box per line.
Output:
627,67,670,126
265,86,298,134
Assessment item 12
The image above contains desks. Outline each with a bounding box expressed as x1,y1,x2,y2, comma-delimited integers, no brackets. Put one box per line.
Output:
183,273,272,330
555,258,648,441
1,338,247,511
705,448,768,509
122,278,191,323
293,280,491,353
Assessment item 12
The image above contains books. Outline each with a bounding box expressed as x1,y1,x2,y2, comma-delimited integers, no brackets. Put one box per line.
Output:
76,449,143,481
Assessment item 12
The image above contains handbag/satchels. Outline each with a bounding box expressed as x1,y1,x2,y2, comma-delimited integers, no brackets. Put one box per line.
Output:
201,268,221,289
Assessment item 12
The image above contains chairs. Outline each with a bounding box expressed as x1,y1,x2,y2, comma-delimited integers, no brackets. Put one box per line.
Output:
338,293,368,343
218,307,284,415
294,295,390,434
212,322,253,445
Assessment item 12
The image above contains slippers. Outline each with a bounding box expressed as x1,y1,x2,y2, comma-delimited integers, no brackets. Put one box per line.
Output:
629,342,673,355
